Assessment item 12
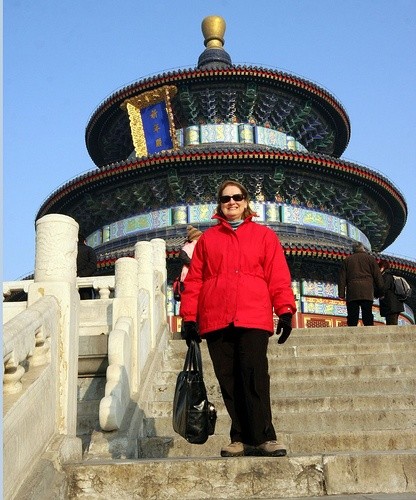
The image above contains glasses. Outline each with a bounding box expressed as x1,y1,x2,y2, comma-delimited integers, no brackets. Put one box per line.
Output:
219,193,244,204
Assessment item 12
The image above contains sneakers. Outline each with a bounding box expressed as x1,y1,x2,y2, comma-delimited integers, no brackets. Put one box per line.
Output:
253,438,287,456
220,440,255,457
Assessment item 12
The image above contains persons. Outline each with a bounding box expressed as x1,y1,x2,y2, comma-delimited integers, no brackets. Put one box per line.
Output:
180,181,298,456
179,226,203,301
339,241,383,326
375,259,406,325
77,233,98,300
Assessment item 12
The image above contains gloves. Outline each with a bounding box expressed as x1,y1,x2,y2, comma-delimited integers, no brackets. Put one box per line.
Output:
183,321,202,348
276,312,293,344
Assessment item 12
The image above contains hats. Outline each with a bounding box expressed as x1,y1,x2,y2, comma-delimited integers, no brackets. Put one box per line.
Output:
185,224,203,242
352,242,364,252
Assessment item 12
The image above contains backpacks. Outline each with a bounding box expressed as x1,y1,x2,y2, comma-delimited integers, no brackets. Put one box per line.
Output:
382,270,413,301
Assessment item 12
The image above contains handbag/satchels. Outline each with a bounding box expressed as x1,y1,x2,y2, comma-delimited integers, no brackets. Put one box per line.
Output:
172,340,217,445
